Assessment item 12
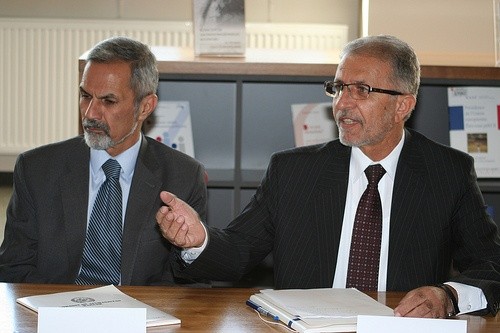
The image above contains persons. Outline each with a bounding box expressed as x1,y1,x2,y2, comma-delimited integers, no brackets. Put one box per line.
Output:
0,39,210,285
155,34,500,319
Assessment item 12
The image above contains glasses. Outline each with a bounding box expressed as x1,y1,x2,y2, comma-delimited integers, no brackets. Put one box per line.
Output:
323,80,404,100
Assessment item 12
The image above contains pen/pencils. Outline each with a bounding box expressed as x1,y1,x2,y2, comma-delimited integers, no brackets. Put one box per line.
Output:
246,300,279,321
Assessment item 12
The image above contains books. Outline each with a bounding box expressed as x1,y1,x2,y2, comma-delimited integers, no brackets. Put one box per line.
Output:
16,284,182,328
247,287,394,333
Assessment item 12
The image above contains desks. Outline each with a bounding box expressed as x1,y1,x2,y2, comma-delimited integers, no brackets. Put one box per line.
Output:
0,282,500,333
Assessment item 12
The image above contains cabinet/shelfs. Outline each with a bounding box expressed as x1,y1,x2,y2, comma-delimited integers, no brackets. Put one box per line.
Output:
77,58,500,286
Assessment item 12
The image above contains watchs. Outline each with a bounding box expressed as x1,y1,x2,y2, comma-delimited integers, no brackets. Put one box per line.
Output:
438,283,460,318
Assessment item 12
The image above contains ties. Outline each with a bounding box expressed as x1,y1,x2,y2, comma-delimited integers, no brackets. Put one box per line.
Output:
346,163,386,292
74,159,122,285
